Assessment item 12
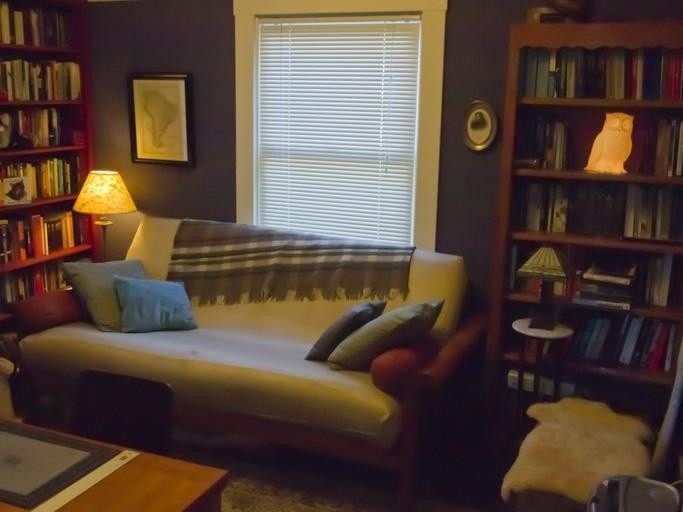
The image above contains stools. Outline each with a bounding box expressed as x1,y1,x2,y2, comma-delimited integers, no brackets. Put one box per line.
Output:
510,320,573,405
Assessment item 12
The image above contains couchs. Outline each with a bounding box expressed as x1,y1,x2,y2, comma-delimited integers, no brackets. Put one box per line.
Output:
7,218,490,502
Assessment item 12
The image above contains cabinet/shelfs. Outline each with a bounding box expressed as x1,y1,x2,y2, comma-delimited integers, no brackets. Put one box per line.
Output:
0,1,103,338
490,22,683,388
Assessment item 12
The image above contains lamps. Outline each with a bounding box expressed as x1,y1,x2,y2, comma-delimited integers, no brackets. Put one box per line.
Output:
516,245,569,332
71,169,138,263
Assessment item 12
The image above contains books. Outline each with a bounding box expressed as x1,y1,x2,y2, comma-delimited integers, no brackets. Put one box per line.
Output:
521,49,682,101
0,107,64,149
514,116,574,172
512,180,682,241
1,265,73,314
573,313,681,377
0,210,89,262
1,1,71,49
0,59,81,102
0,154,81,204
651,117,683,177
568,250,682,309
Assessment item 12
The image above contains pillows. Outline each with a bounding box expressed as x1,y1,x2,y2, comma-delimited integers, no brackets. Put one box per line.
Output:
327,297,446,370
112,275,199,333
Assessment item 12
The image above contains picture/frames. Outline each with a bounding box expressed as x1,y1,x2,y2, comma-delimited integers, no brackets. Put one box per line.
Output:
464,100,496,153
124,72,192,169
